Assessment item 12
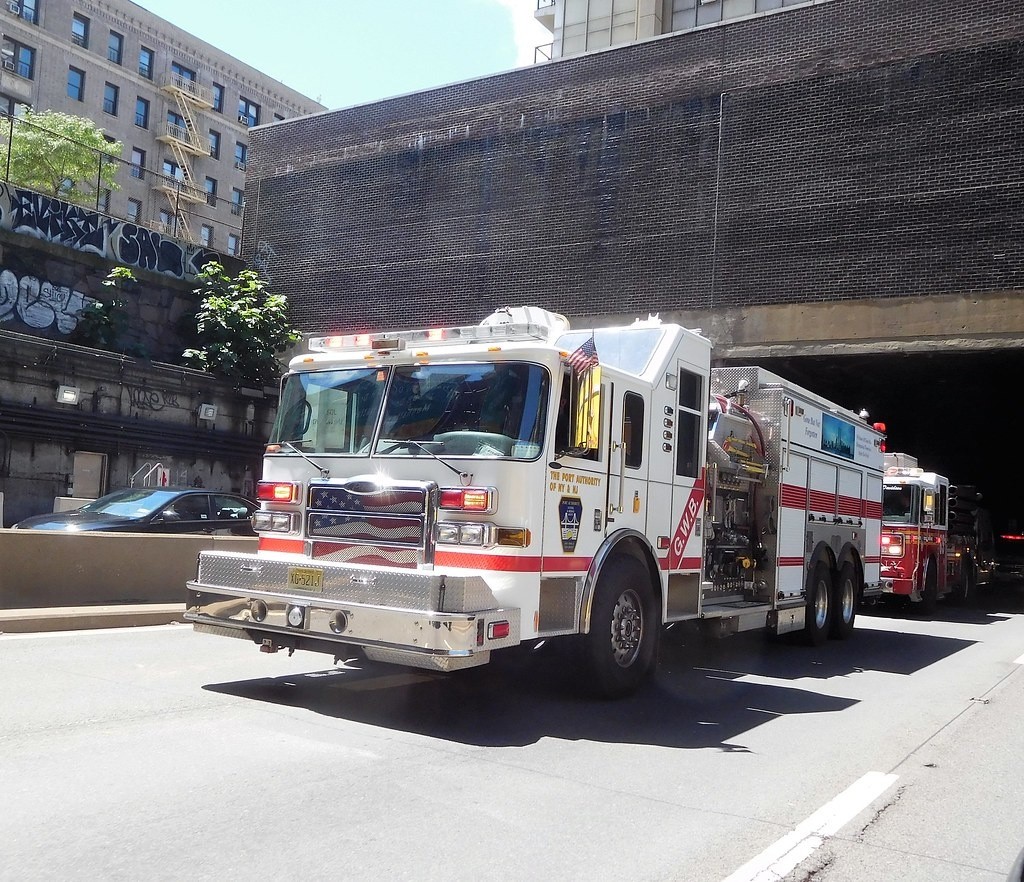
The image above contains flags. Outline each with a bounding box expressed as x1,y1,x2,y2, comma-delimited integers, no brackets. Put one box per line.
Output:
566,337,599,374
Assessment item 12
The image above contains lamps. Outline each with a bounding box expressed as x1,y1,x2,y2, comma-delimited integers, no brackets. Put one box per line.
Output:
197,403,219,421
55,385,81,406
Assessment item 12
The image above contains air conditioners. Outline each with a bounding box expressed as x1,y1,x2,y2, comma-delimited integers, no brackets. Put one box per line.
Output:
238,115,249,124
9,3,20,14
2,60,15,71
235,162,245,171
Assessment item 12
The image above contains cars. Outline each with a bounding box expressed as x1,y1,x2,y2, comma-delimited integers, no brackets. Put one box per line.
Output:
8,484,264,538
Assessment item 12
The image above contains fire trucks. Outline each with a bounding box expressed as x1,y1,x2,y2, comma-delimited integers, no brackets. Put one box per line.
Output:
879,451,999,616
179,307,890,703
